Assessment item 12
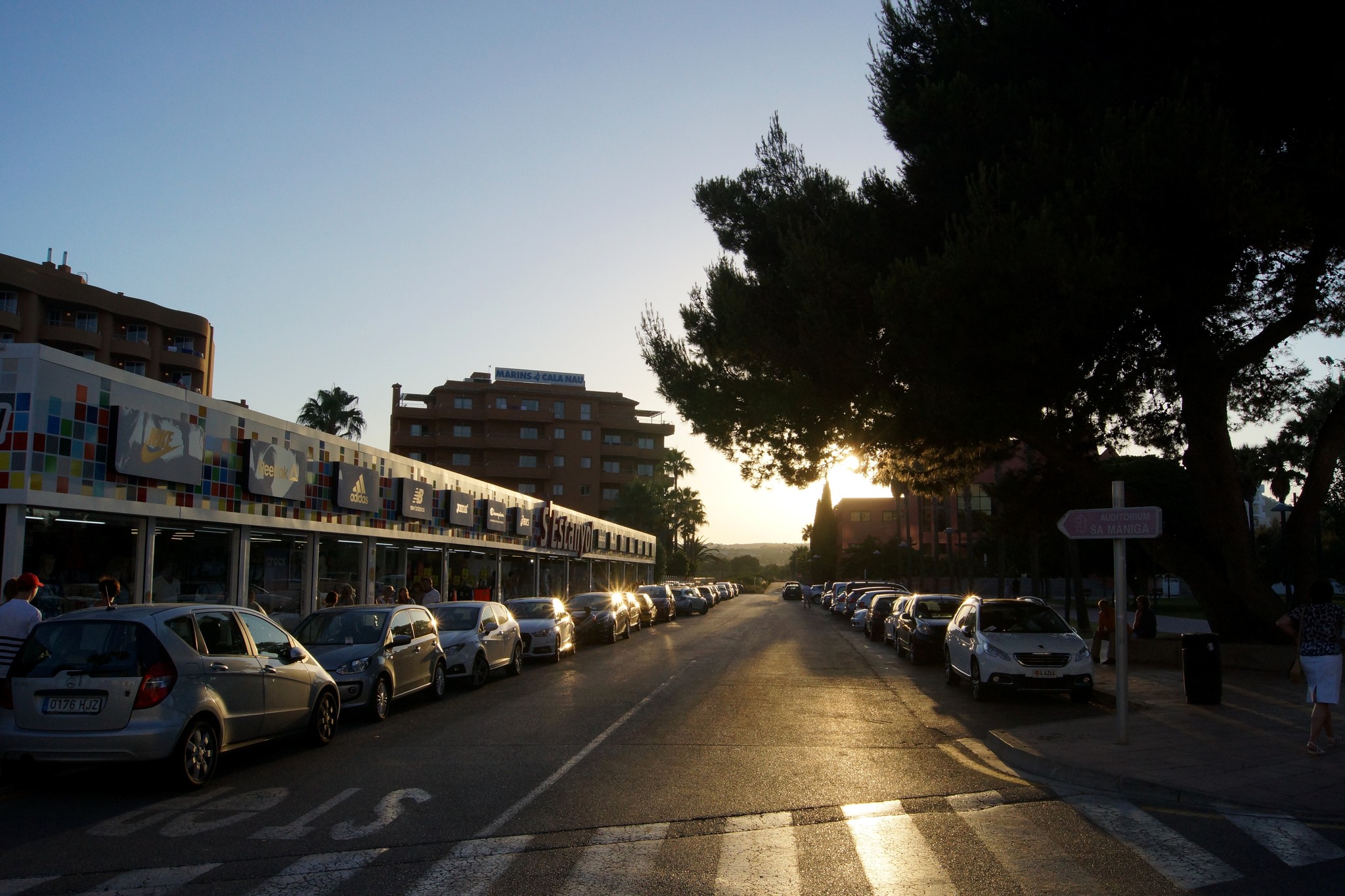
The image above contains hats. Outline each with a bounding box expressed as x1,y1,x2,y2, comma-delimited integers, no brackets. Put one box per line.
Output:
16,572,44,587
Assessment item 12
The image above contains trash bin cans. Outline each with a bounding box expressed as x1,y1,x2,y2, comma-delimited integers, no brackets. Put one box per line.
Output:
1181,632,1224,705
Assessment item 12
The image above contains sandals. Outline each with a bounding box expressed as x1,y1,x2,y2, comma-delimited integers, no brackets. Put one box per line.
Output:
1327,735,1342,743
1307,741,1325,755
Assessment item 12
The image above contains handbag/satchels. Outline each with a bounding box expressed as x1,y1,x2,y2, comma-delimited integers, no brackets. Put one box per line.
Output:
1288,659,1301,685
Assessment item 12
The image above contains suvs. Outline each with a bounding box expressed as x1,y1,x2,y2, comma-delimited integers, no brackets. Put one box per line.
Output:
634,585,677,622
715,582,735,599
942,595,1098,703
809,581,909,627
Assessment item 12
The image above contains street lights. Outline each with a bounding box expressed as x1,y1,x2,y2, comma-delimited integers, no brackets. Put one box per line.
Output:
781,542,797,581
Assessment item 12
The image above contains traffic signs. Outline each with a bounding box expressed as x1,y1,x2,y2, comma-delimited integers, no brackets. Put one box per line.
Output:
1056,507,1165,541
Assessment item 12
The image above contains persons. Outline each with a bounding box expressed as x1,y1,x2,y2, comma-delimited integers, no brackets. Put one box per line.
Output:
1004,575,1020,599
1090,599,1135,665
802,583,812,609
1276,580,1345,756
1131,595,1157,639
0,567,621,677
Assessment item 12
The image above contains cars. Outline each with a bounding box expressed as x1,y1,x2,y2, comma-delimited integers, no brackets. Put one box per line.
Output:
704,585,722,604
293,603,449,722
499,598,577,664
782,581,803,600
633,594,659,628
862,594,913,642
717,585,729,601
670,586,708,616
883,596,914,646
857,590,914,610
896,594,965,666
737,584,744,594
730,584,739,597
693,586,715,607
1,603,341,790
615,592,642,631
423,601,524,688
562,593,631,644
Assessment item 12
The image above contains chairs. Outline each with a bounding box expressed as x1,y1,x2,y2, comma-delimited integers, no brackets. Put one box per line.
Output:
659,590,666,598
542,605,551,614
469,609,479,619
336,621,379,644
919,603,928,613
879,603,890,609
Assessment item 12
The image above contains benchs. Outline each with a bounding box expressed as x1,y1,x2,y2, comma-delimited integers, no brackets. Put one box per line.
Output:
1150,588,1163,599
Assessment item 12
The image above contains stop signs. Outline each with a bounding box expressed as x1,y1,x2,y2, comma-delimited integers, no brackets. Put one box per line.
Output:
797,574,802,579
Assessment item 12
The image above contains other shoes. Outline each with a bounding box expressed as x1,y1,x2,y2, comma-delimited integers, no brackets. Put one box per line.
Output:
1092,656,1100,663
1102,659,1114,665
